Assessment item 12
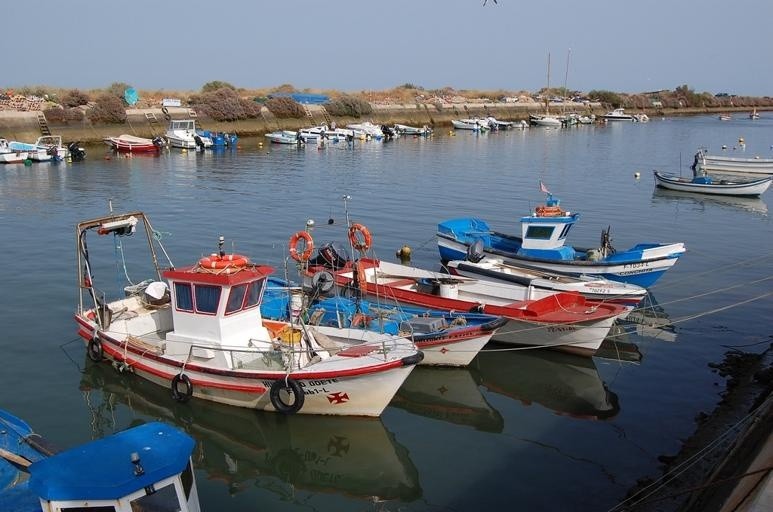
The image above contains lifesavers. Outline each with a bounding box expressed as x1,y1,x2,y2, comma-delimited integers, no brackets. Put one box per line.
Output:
87,337,102,362
171,374,194,403
289,231,314,261
356,260,367,297
348,224,372,251
340,281,357,299
199,253,249,270
270,378,305,415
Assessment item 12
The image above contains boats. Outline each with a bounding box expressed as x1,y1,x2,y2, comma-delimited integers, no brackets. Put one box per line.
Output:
697,149,773,177
72,198,425,417
600,108,639,123
530,118,567,127
719,112,732,120
598,325,642,366
475,341,621,423
298,125,354,142
164,120,213,148
346,122,401,142
102,134,165,153
0,137,29,162
389,123,432,135
656,189,767,215
435,180,687,324
265,130,308,144
82,356,426,503
578,116,593,124
8,135,87,161
451,117,515,130
626,292,677,343
653,169,773,194
259,271,509,367
750,112,760,120
1,411,200,512
392,361,505,435
449,240,646,323
529,113,567,124
297,195,623,358
